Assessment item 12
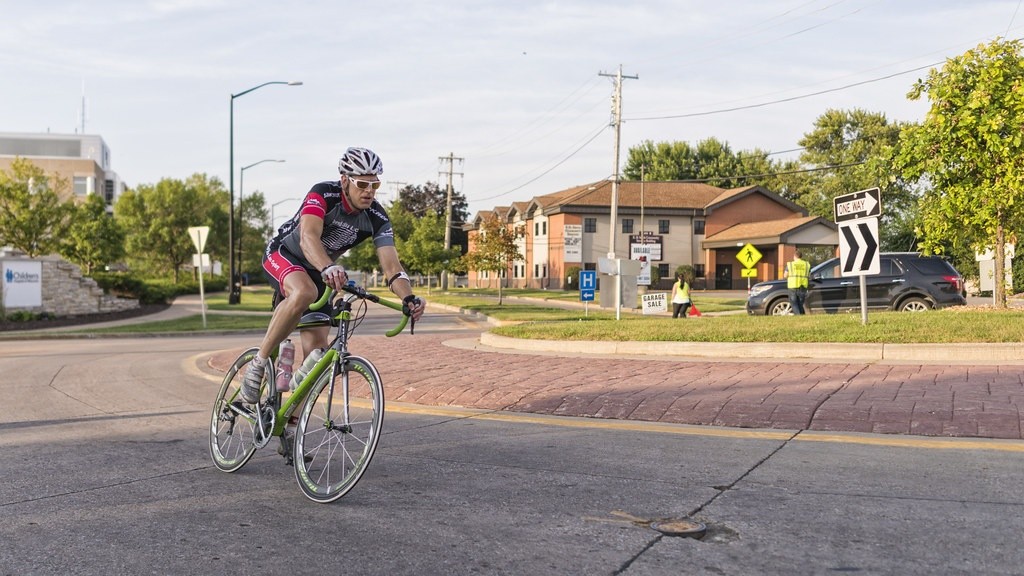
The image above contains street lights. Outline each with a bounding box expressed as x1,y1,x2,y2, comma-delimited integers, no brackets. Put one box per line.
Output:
228,79,303,310
237,158,303,308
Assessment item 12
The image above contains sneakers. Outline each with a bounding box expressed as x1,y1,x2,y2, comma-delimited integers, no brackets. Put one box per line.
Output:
240,360,265,405
277,435,314,463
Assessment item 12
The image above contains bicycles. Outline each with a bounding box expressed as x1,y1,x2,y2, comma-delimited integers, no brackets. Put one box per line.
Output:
207,268,422,501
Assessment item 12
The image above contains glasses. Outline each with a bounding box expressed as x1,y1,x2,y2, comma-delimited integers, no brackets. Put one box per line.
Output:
345,175,381,190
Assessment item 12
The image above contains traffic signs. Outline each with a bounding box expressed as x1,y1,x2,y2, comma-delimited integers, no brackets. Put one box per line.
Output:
834,184,881,222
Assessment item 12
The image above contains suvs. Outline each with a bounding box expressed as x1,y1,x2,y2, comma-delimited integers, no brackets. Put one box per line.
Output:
743,250,971,317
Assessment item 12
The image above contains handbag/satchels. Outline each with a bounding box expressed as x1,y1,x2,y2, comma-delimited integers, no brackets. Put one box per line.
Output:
688,299,693,307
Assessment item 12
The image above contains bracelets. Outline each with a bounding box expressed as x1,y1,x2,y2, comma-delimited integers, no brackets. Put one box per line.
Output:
321,264,336,272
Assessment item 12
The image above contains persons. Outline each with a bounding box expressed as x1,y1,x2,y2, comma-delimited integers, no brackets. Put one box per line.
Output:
240,146,427,462
784,251,811,315
671,274,691,318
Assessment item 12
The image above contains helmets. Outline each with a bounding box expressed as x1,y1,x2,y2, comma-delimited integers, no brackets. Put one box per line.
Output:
338,147,383,175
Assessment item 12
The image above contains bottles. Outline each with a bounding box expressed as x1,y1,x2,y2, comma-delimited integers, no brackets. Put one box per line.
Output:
289,348,324,390
277,339,295,391
